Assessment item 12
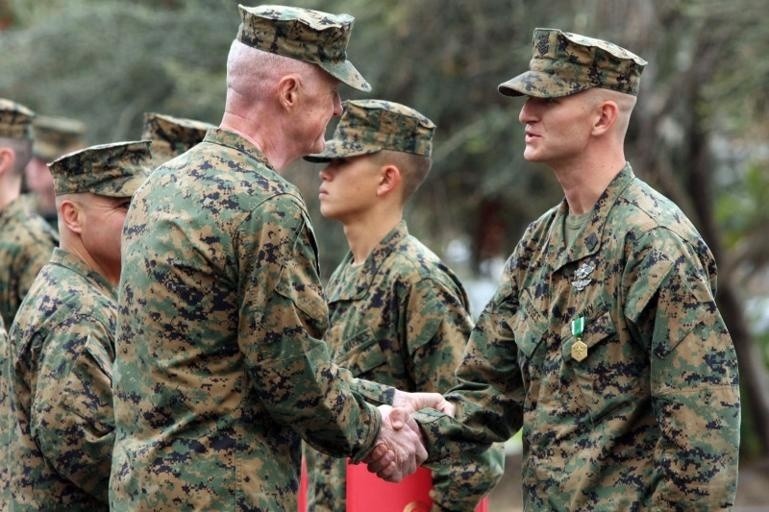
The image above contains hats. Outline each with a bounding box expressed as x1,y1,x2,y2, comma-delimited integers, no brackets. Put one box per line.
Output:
47,140,159,199
235,1,373,93
1,93,38,143
140,108,222,161
496,27,649,101
29,109,91,164
300,96,438,162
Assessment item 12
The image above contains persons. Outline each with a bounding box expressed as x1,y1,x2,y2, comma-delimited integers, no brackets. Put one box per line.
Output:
363,23,743,510
296,97,508,510
104,3,450,511
